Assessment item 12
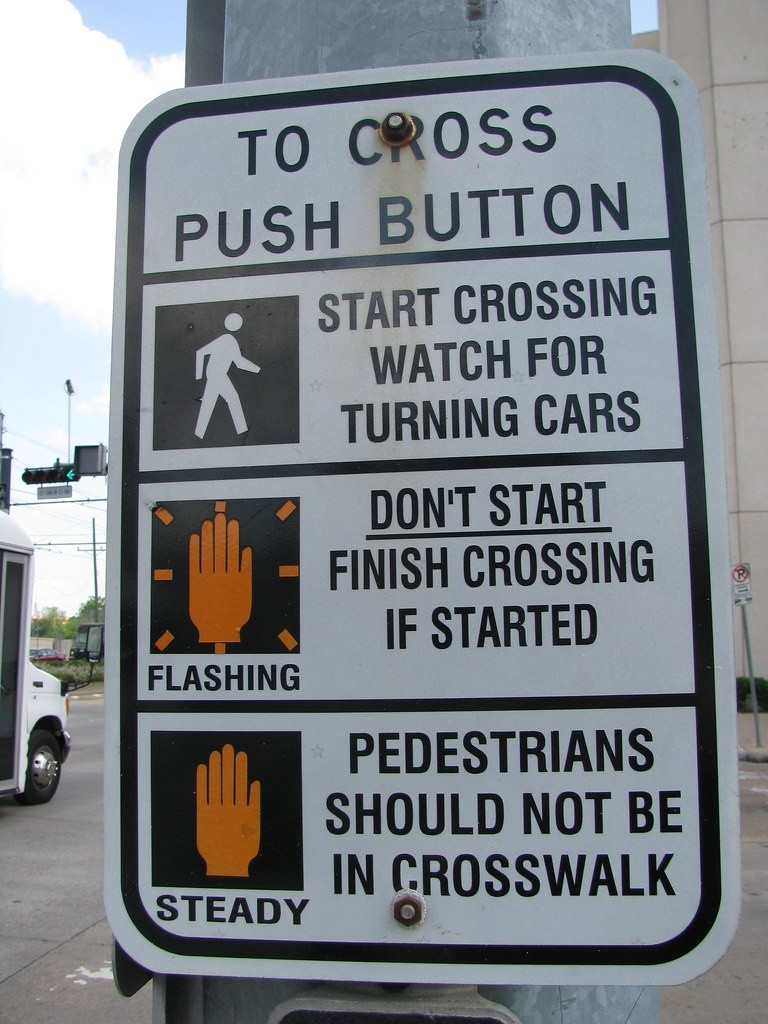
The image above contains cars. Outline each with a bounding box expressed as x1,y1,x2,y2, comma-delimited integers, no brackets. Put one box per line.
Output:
28,647,68,662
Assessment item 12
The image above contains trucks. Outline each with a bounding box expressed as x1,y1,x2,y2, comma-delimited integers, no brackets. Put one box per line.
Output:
0,509,105,808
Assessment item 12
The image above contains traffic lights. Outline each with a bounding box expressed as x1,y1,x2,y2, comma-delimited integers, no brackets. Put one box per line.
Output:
20,465,83,487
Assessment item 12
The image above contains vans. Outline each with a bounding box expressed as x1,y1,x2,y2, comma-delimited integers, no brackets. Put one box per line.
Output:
69,623,103,659
